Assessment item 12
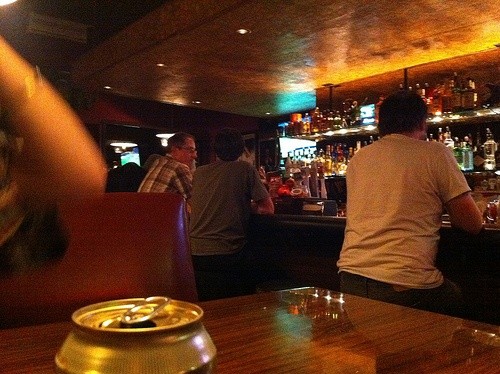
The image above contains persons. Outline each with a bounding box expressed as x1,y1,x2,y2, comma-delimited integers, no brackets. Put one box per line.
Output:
137,132,197,214
189,129,274,302
0,34,107,275
336,90,483,319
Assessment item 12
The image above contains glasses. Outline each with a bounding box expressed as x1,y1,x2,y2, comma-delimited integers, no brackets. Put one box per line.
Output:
181,147,195,153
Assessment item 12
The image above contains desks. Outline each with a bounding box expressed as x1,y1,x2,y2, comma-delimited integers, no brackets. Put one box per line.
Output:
1,287,500,374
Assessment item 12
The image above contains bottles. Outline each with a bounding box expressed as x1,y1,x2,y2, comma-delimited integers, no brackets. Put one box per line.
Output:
286,135,377,176
428,124,499,171
398,72,478,115
286,100,362,135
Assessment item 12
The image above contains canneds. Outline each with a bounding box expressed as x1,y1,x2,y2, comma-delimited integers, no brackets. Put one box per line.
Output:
54,296,218,373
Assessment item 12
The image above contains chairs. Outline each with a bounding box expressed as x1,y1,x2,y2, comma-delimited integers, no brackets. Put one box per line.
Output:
0,192,198,330
